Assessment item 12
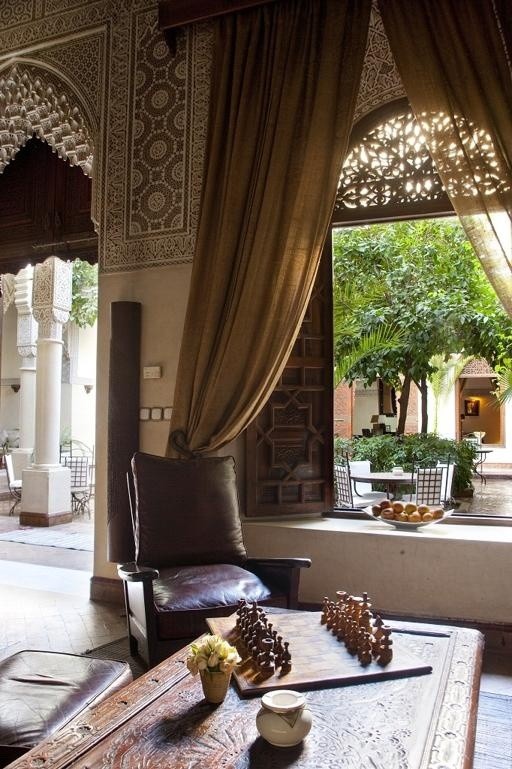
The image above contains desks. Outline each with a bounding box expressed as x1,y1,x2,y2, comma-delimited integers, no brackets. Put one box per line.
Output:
349,471,418,502
473,449,494,484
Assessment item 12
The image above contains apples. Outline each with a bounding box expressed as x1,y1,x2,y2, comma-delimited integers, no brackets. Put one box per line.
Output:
383,507,394,518
392,500,403,514
433,507,444,519
409,511,421,521
422,513,432,520
404,503,416,513
418,504,428,514
396,512,406,520
380,499,391,510
373,505,380,515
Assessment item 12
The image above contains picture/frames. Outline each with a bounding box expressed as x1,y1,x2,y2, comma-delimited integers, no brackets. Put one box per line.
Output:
464,399,480,416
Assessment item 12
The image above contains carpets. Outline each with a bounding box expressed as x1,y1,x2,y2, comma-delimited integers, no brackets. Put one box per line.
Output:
82,635,512,767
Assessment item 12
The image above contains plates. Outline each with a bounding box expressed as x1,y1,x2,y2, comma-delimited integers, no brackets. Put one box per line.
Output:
363,504,455,533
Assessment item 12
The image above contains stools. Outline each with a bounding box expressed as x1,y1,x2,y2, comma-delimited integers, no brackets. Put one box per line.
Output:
0,649,133,765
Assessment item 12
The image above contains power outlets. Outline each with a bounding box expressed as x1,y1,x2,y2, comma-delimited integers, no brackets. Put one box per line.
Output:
143,366,161,379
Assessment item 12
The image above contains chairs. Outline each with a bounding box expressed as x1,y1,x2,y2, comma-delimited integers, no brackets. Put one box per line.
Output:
334,448,394,508
403,452,450,509
3,454,22,515
116,472,312,671
59,455,94,519
380,424,386,434
362,429,371,437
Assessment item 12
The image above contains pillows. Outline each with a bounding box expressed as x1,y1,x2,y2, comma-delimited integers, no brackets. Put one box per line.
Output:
131,450,247,563
